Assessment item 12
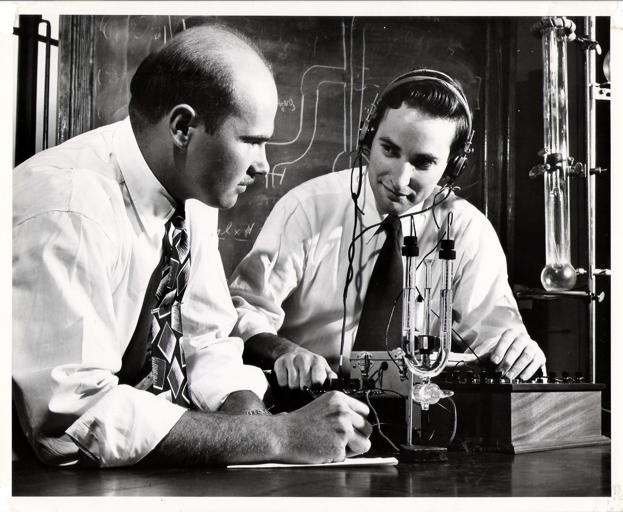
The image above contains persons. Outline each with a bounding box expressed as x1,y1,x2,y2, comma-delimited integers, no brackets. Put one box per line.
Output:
227,68,548,390
11,25,372,468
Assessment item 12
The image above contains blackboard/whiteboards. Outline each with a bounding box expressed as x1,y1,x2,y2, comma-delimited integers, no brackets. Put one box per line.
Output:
94,20,482,317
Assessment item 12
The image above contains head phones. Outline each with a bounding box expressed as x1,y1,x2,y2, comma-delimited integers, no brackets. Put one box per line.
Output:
358,69,476,182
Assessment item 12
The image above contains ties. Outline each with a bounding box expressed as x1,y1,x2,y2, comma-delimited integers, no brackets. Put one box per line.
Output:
352,214,404,350
134,203,192,409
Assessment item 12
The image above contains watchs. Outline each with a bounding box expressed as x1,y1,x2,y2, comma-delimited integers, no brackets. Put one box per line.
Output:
242,409,273,416
